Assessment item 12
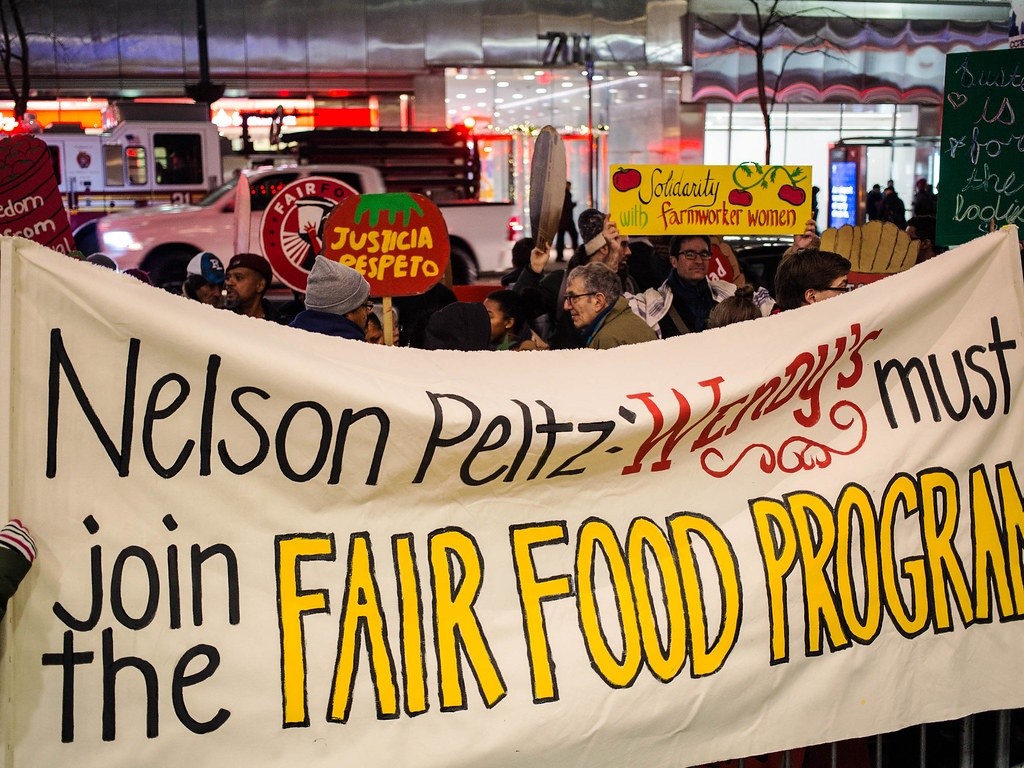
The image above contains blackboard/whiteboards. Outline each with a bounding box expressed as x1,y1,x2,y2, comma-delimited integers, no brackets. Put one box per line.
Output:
934,47,1024,246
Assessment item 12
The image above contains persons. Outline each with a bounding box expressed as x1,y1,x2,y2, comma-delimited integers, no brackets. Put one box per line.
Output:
0,520,37,622
78,146,940,350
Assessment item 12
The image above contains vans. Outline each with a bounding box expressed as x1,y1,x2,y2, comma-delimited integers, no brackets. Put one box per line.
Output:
36,108,223,232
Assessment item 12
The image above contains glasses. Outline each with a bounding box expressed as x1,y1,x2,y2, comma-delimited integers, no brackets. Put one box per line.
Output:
563,291,598,305
910,233,921,240
678,250,712,260
809,284,854,292
361,305,373,313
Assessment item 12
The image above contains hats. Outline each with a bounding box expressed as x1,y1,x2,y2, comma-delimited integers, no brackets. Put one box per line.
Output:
578,209,606,243
885,186,894,191
226,253,272,288
123,269,151,286
68,251,85,260
187,251,226,283
85,252,116,270
303,256,370,315
628,237,653,248
917,179,926,187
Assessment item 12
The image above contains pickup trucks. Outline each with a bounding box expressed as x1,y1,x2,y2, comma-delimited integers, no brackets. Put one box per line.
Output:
95,166,521,297
